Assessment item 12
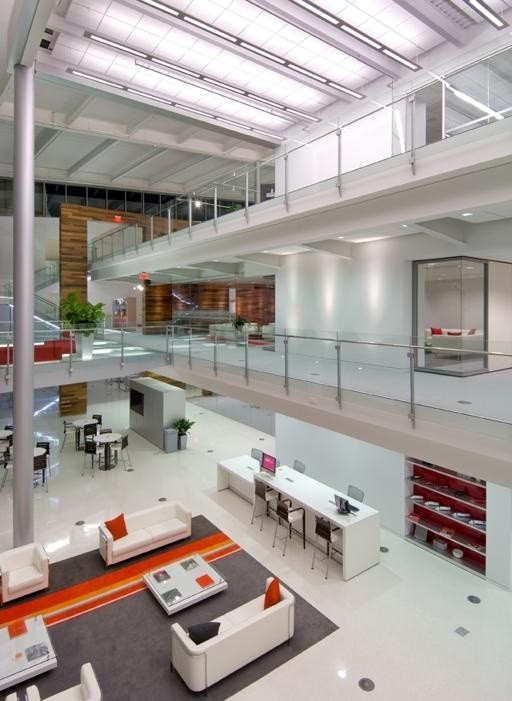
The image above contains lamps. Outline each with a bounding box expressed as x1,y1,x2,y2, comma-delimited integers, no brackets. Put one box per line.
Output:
127,87,173,104
251,127,286,140
383,47,421,69
329,79,364,99
286,106,319,122
138,0,180,16
290,0,340,23
248,92,284,108
465,0,505,27
340,23,383,50
289,61,328,82
90,33,149,57
183,14,238,42
203,76,246,93
215,115,252,129
240,40,287,63
72,67,124,89
151,56,202,77
174,102,216,117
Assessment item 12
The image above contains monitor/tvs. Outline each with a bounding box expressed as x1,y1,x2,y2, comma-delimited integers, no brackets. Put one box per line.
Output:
335,494,349,513
261,452,276,476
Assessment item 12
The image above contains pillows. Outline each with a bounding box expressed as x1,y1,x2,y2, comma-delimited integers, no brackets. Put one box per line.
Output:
104,513,128,541
263,577,281,610
188,621,221,644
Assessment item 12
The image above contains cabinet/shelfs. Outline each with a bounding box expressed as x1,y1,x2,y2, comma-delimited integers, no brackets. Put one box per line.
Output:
404,455,486,578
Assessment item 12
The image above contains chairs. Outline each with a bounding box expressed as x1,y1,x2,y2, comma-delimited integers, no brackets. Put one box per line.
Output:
348,485,364,502
110,433,132,471
252,479,283,530
293,459,305,473
92,414,102,430
5,425,15,431
59,420,78,454
273,498,306,556
9,434,13,447
2,446,12,486
311,514,342,580
36,441,52,480
81,441,103,477
100,428,113,463
84,423,98,442
34,454,48,493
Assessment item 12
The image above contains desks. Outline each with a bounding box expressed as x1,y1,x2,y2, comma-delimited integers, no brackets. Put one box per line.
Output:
0,429,13,439
216,455,381,581
72,419,99,451
10,446,46,458
93,432,121,471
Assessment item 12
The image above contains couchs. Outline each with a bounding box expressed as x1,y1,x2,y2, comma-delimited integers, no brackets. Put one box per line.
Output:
98,500,192,566
0,542,50,606
1,661,102,701
425,324,485,360
208,321,257,337
260,321,273,338
170,577,296,698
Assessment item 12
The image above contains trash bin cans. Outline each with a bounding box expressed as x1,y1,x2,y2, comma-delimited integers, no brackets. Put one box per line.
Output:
164,428,178,453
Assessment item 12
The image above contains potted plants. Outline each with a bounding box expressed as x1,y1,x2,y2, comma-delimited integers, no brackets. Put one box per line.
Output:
173,415,196,449
233,313,251,342
60,290,106,360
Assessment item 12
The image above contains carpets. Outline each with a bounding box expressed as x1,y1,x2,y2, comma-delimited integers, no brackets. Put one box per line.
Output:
1,513,339,701
